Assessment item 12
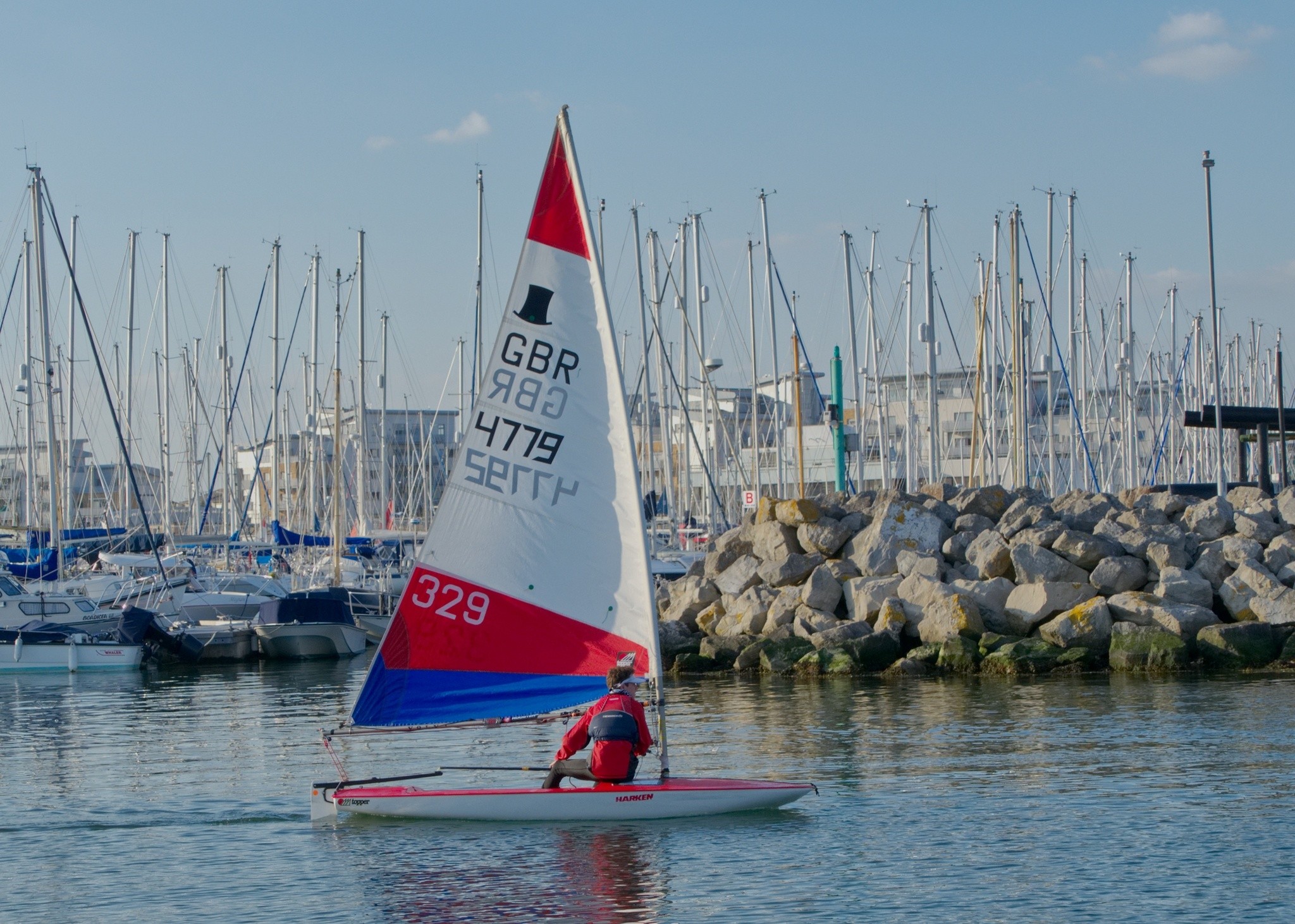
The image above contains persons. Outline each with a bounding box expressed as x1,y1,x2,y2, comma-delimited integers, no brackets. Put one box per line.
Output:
541,666,651,789
683,510,696,538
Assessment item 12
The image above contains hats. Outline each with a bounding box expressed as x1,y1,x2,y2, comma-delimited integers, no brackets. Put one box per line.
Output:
608,673,646,693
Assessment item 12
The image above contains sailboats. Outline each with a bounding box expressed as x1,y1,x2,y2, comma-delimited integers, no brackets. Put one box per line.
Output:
307,103,720,827
1,147,755,667
